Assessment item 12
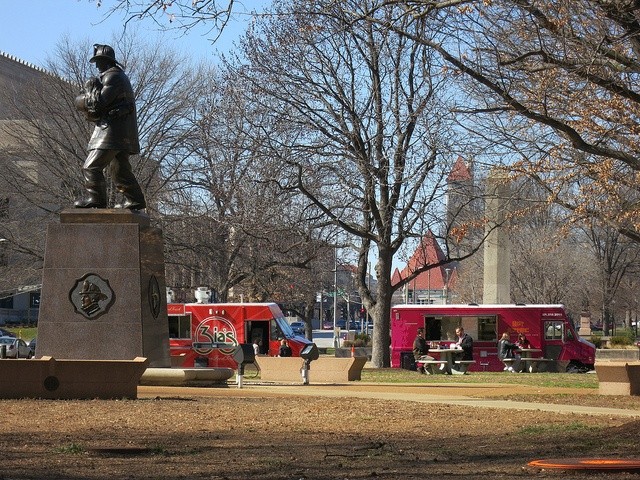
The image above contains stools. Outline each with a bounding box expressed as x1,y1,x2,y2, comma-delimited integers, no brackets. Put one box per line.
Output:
502,352,526,371
416,353,446,375
521,352,553,373
446,353,476,375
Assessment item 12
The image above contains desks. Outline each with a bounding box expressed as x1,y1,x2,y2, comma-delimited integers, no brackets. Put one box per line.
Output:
516,349,542,358
428,349,463,361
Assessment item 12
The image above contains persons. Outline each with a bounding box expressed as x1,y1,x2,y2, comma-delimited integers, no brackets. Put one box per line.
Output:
452,327,473,370
278,339,292,357
513,333,535,372
498,332,519,372
413,327,437,374
73,43,146,209
252,339,260,355
79,280,108,316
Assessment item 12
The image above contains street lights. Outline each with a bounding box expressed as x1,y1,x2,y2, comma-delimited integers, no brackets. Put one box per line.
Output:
443,267,452,304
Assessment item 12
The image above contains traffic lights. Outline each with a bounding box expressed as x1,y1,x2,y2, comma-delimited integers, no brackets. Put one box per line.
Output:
359,308,366,319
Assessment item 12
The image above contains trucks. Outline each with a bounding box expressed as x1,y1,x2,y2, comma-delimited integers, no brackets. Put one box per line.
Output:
165,286,312,370
390,302,596,372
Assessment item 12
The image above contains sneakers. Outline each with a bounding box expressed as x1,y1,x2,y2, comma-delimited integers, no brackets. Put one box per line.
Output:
417,367,425,375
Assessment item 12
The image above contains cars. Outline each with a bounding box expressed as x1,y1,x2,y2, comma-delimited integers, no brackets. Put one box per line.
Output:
29,338,37,355
323,320,374,330
290,322,305,335
0,328,16,337
0,337,32,359
